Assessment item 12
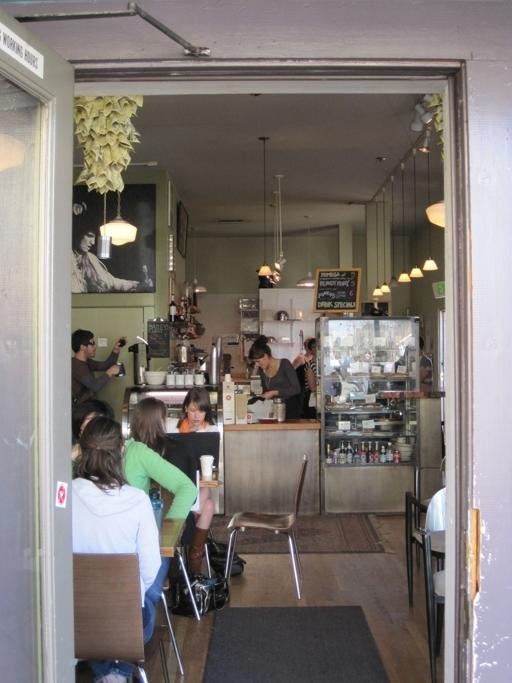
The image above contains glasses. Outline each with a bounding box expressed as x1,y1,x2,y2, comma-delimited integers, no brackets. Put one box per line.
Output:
86,341,95,345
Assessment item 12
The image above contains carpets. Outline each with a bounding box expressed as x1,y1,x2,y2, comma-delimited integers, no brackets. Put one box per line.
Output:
200,605,391,682
207,511,392,554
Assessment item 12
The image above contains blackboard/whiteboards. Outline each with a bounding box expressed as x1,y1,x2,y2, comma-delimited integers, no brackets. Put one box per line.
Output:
313,268,362,313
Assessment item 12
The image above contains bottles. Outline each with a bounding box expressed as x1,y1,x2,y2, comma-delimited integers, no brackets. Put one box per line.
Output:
360,441,367,463
169,292,177,321
273,396,287,422
353,443,360,463
393,449,400,464
380,445,386,463
332,449,338,463
325,444,332,463
346,440,353,463
338,440,346,463
150,492,164,546
367,441,374,462
386,441,393,463
373,441,380,463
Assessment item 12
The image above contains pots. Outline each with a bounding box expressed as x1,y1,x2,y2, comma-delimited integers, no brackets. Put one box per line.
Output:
373,418,401,430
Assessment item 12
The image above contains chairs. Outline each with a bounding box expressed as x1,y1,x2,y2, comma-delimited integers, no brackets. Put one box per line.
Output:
73,551,172,682
417,526,446,682
221,454,309,601
410,463,434,530
150,478,202,620
403,490,429,608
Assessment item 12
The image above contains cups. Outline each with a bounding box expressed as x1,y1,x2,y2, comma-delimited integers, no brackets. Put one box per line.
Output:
115,360,126,376
185,374,194,388
195,373,204,385
175,374,184,388
166,374,175,388
200,454,215,481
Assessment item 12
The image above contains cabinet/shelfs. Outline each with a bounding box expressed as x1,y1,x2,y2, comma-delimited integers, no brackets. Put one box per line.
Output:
259,296,303,345
315,315,420,516
120,384,227,517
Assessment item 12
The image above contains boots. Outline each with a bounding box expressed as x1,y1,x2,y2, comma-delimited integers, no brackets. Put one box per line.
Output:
188,529,208,574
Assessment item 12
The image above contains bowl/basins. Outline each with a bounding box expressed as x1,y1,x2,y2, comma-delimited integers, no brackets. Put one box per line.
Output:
143,371,167,384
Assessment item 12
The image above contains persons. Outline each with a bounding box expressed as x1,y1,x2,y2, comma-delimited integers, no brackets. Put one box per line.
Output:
304,338,319,420
171,385,220,537
71,221,142,292
72,414,161,682
71,327,129,412
72,397,198,610
419,336,435,392
126,395,198,616
290,337,311,418
248,334,303,419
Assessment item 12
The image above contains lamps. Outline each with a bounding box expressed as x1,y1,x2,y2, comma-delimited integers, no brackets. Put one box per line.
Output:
397,162,412,282
191,225,208,292
258,135,272,276
295,215,317,287
269,175,287,284
379,185,391,292
98,189,138,247
424,199,446,229
417,120,433,153
373,197,384,295
409,148,424,277
387,174,399,288
422,150,438,271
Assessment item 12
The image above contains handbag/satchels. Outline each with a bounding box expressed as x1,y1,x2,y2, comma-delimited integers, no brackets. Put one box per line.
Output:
172,575,229,616
209,542,246,577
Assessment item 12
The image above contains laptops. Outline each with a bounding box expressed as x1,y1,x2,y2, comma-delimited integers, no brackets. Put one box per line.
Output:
163,431,221,476
